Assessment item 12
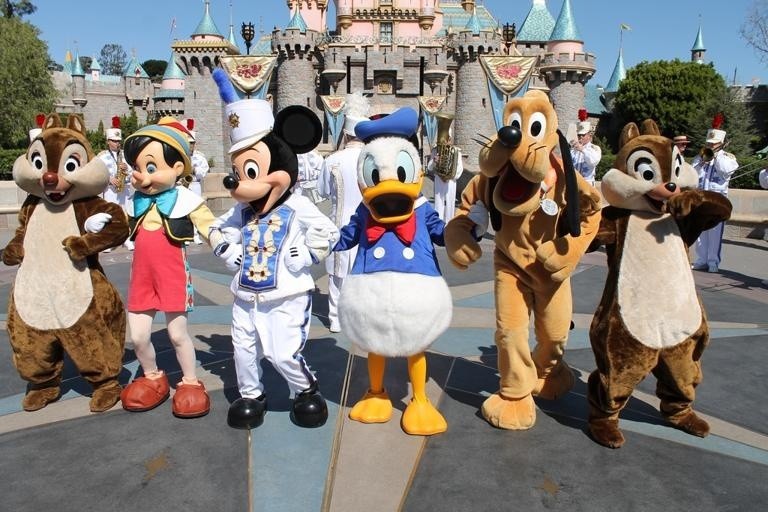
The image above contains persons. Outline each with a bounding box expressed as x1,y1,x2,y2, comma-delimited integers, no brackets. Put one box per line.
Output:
97,128,135,253
184,132,209,245
673,135,691,152
690,129,740,273
290,150,326,204
568,121,602,188
428,129,464,223
317,113,371,334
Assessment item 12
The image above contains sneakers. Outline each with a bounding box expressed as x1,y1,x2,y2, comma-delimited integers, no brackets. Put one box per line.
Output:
121,240,136,251
190,236,204,245
708,265,718,273
691,264,708,270
102,246,116,253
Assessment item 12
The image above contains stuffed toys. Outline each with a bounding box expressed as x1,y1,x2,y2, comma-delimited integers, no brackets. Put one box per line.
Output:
0,111,129,412
207,99,341,429
558,117,732,449
443,88,602,431
84,116,241,419
305,105,489,436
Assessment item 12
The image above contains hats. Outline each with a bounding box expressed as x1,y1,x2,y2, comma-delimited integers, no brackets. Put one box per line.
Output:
185,130,197,143
674,135,691,144
343,114,370,137
576,121,591,135
106,128,122,142
29,127,45,143
706,129,726,144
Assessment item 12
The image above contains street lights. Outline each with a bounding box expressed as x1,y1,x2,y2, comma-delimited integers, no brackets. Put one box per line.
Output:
241,21,254,55
501,20,517,55
